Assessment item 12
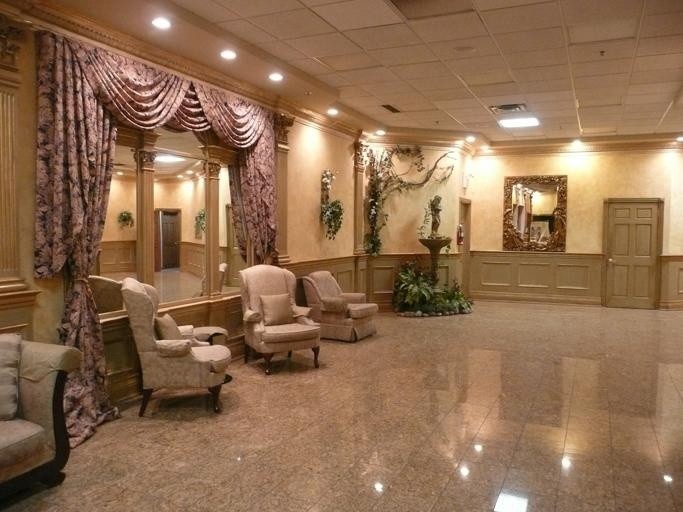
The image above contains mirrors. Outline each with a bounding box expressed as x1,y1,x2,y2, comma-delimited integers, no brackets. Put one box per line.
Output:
503,175,567,252
88,120,260,331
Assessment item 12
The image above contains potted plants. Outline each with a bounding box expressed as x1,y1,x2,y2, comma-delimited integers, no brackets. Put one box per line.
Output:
117,211,135,230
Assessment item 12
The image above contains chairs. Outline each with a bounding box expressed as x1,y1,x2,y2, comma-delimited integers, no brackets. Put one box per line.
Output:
302,270,378,342
120,277,232,417
0,341,84,512
193,262,227,299
238,265,321,375
95,249,102,275
87,275,123,314
530,221,550,244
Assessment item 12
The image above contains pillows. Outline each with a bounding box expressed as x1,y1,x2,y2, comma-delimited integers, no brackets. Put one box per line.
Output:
155,313,183,339
260,293,296,325
0,334,21,421
